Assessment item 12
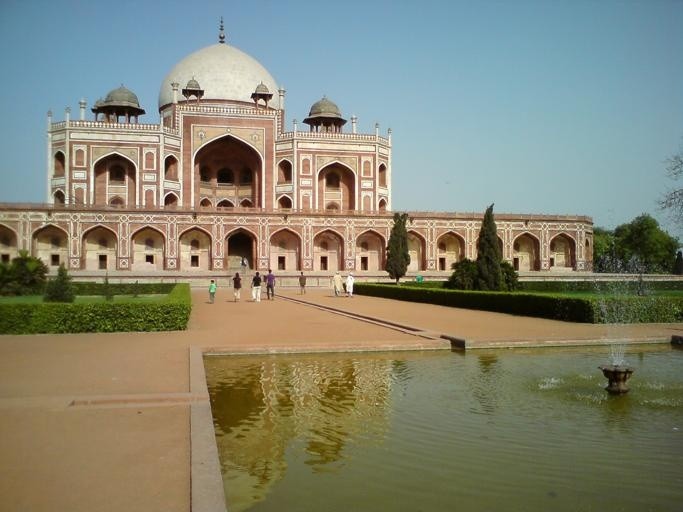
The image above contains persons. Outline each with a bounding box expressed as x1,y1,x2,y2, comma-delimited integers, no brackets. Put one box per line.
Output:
231,272,242,303
344,273,355,298
250,271,261,302
207,279,216,303
332,272,344,297
239,256,249,274
264,269,275,301
297,271,306,295
